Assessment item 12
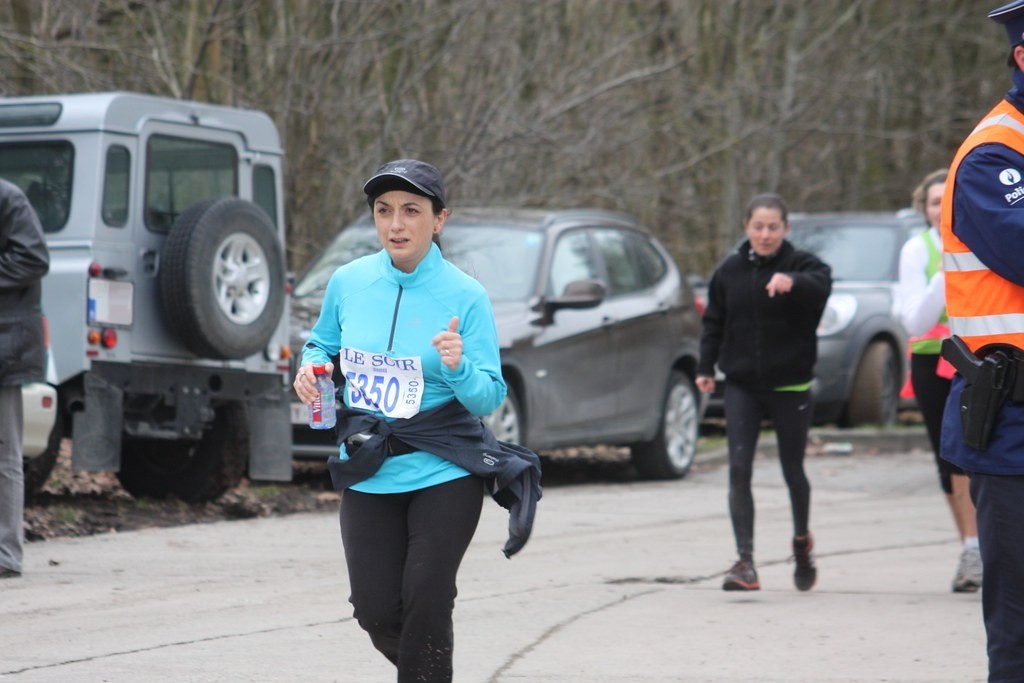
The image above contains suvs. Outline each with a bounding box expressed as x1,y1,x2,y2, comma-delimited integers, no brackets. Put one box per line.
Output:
703,209,928,429
1,91,297,507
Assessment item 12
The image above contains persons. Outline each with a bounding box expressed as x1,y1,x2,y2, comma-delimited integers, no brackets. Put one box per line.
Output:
0,178,50,578
695,194,833,590
898,0,1024,683
292,160,508,683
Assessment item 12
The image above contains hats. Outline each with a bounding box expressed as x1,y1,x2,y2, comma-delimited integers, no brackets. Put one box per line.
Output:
363,159,445,206
987,0,1024,48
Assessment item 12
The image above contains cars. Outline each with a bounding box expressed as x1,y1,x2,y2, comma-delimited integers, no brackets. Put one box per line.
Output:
285,203,707,494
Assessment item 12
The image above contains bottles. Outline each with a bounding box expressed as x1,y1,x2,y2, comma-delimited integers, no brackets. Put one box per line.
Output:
307,364,336,429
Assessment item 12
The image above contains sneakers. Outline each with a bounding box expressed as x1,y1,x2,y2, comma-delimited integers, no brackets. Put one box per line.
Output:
952,546,983,591
786,531,818,590
723,561,761,590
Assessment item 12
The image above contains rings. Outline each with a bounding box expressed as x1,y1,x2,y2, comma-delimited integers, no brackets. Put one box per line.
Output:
299,373,306,382
445,349,450,355
